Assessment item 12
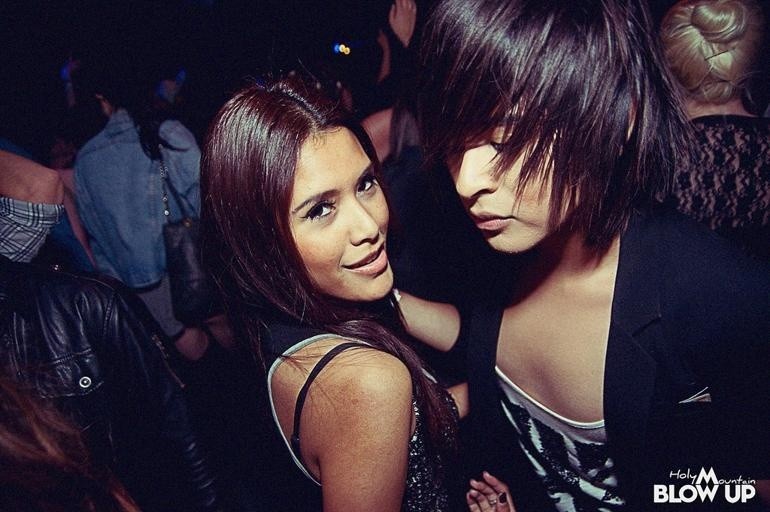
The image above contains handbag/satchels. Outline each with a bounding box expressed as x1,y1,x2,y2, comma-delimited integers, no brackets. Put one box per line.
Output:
146,127,224,324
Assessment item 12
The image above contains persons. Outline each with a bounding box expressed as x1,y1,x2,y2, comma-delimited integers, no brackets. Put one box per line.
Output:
0,0,770,512
